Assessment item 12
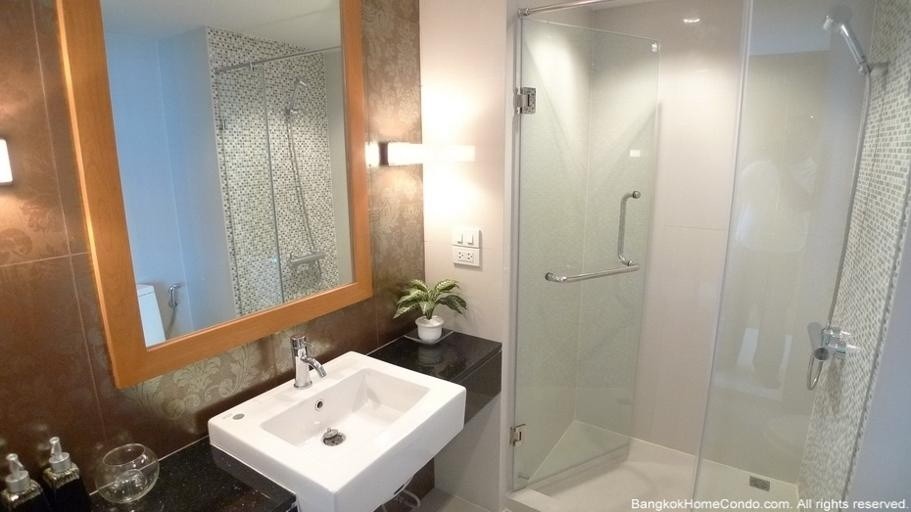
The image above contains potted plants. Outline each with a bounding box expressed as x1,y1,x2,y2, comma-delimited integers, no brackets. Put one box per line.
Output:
392,279,469,345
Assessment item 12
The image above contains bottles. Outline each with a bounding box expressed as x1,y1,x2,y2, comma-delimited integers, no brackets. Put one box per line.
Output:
95,444,160,507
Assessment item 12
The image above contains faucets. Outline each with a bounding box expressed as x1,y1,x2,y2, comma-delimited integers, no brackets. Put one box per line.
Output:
289,333,327,389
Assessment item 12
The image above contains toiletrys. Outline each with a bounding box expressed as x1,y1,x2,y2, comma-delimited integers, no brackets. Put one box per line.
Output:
41,436,93,512
0,453,53,512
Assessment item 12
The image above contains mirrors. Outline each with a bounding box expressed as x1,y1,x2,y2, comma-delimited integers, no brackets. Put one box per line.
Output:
57,1,373,390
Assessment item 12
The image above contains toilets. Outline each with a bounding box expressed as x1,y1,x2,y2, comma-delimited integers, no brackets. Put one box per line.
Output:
135,283,166,348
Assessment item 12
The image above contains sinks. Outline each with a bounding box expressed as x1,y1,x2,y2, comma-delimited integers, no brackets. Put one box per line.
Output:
207,349,468,512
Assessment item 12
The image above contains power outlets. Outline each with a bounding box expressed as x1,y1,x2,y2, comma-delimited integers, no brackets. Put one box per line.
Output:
451,245,479,267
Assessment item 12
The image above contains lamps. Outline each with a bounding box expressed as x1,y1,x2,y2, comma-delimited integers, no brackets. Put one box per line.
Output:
0,138,13,188
378,139,431,167
